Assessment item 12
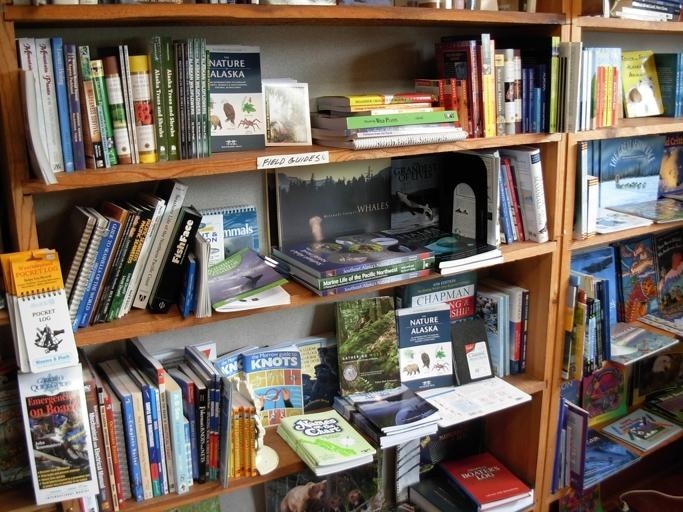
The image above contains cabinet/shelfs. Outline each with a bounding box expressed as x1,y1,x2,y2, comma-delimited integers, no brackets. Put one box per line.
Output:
540,22,683,512
0,7,570,512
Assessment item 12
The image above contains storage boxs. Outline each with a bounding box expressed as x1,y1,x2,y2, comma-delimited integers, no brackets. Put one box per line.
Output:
571,247,619,325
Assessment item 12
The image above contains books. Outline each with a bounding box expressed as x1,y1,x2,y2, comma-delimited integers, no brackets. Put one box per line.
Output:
31,182,292,324
14,37,312,184
173,431,535,512
561,235,683,380
77,343,376,512
292,273,531,475
0,251,99,510
551,349,683,496
15,0,311,6
263,146,550,295
574,133,683,241
572,0,683,128
312,0,572,150
